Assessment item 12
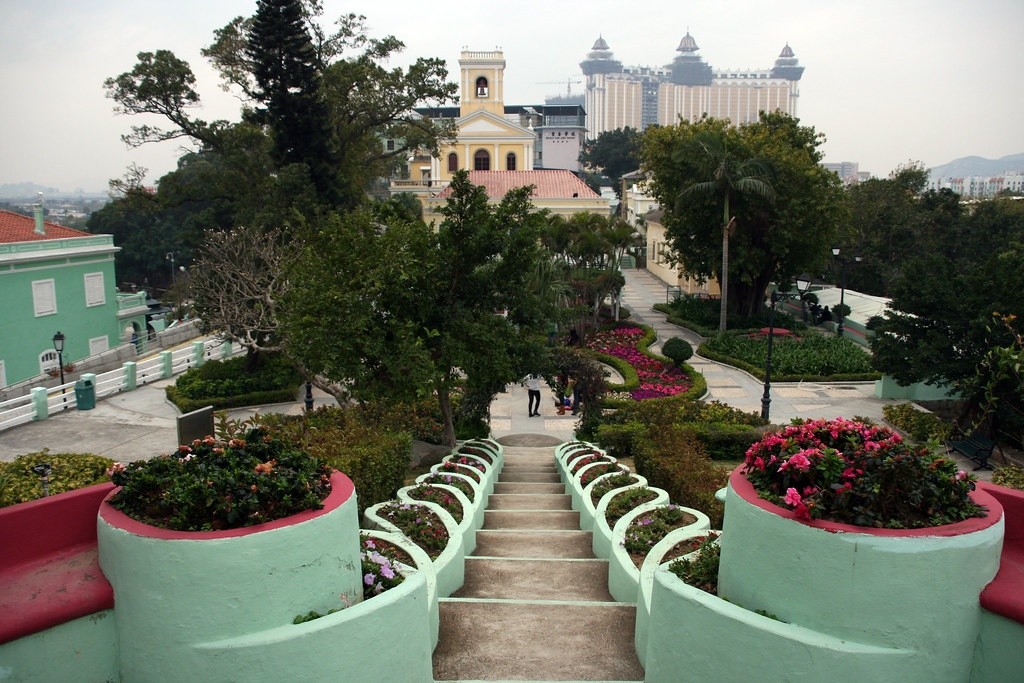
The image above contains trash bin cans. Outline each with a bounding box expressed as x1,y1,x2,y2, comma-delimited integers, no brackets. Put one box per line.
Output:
74,380,97,410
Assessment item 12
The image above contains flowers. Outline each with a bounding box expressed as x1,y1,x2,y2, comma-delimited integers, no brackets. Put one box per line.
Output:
557,326,693,399
560,439,719,565
355,448,486,601
106,421,331,532
742,417,994,530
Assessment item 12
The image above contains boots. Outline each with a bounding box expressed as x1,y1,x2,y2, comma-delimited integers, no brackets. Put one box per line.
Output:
556,405,565,415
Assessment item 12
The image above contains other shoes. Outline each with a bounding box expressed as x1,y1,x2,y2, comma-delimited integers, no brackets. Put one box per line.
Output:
529,413,534,417
533,412,541,416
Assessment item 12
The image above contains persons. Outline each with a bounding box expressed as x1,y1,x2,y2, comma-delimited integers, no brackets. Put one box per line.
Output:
131,333,139,356
571,380,583,415
771,290,778,309
177,317,183,325
523,373,542,417
555,372,568,415
809,304,832,326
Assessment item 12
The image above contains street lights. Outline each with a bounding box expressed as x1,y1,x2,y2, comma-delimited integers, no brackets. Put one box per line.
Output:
831,244,847,337
165,251,176,288
52,330,68,410
